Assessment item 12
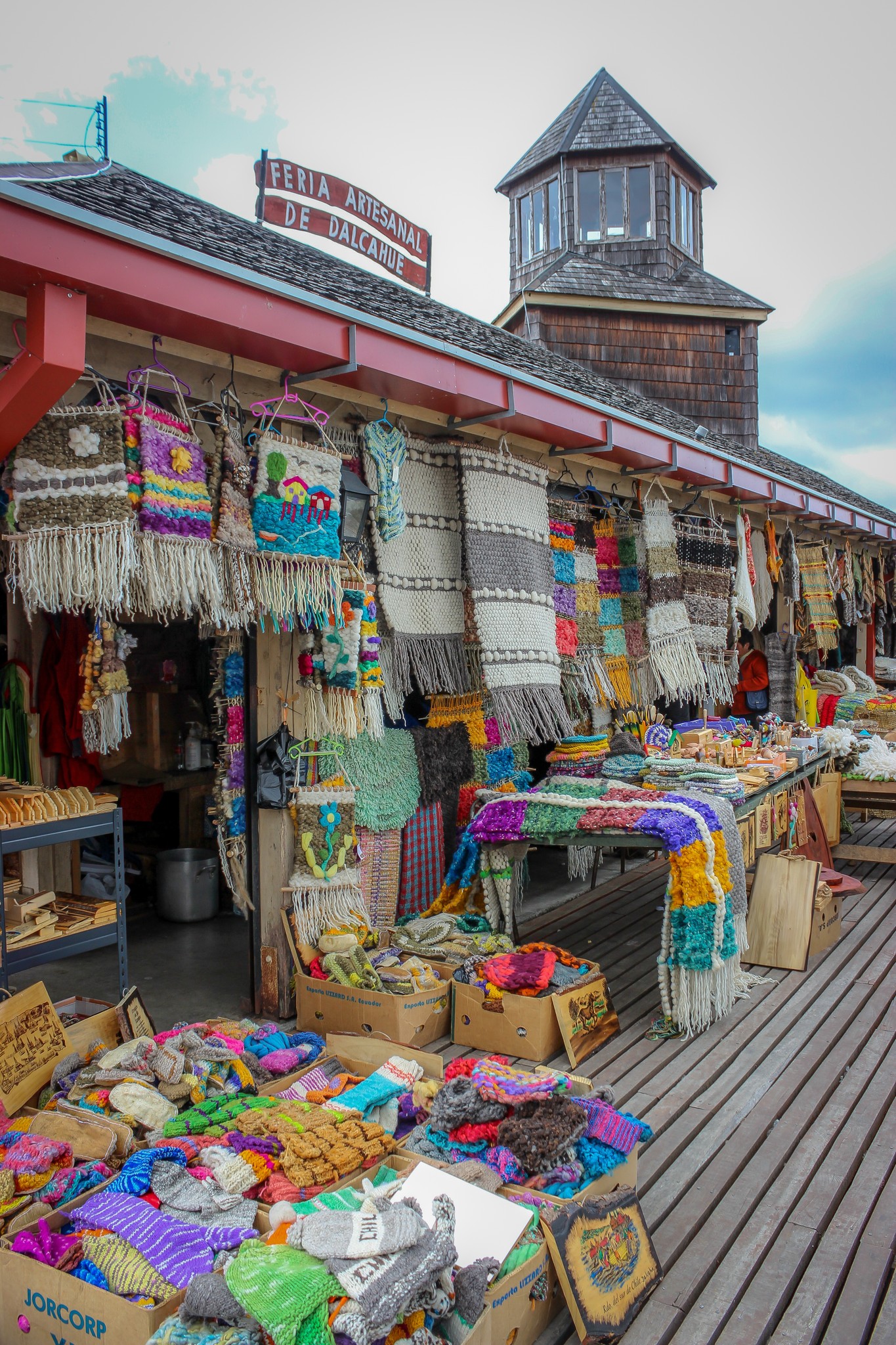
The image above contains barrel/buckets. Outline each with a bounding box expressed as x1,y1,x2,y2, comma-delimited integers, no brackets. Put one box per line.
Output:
155,848,219,923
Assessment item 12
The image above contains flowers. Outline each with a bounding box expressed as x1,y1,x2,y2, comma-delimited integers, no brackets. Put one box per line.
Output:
735,499,790,531
796,518,885,561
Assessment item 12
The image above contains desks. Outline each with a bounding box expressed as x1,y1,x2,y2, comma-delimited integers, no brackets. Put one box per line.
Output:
481,757,831,945
829,780,896,866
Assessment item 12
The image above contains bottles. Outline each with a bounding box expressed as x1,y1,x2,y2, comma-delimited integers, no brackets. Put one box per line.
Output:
186,729,201,770
173,728,185,770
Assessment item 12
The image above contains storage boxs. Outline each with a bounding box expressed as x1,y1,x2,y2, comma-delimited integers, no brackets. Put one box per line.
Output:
673,717,825,779
0,947,641,1345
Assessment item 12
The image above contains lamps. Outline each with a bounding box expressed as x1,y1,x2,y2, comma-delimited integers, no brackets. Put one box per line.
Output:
335,464,381,570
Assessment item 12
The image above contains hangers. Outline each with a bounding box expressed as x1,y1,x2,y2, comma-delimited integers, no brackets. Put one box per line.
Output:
375,398,393,430
777,623,801,642
0,319,330,452
550,458,729,540
288,733,344,760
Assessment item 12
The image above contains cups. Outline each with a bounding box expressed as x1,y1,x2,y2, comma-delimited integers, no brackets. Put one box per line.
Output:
200,744,215,767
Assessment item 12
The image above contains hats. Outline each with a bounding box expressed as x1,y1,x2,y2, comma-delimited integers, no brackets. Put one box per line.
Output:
178,1187,498,1345
601,733,648,780
453,942,591,1013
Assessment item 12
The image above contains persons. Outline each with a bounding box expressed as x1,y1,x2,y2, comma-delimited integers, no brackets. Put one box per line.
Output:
728,628,769,731
795,650,816,685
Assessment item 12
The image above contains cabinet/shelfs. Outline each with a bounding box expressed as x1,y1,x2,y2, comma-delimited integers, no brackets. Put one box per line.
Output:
0,807,127,1001
72,674,220,918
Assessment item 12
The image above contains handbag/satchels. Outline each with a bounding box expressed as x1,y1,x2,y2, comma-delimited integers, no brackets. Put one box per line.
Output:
746,689,768,711
795,663,818,728
